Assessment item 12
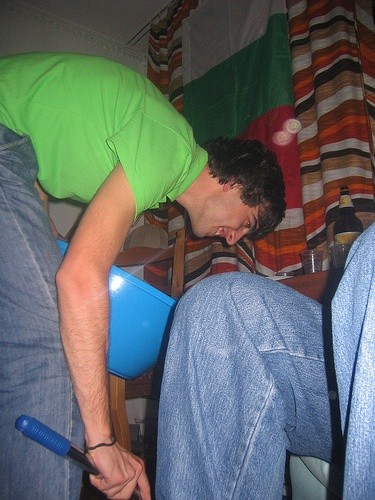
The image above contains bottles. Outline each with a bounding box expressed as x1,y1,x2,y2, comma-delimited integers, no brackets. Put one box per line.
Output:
333,185,364,244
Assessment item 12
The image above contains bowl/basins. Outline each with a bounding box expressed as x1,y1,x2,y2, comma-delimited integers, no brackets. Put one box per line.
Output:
53,236,178,380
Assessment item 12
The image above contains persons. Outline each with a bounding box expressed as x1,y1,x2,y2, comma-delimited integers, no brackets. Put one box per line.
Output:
0,51,286,500
155,222,375,500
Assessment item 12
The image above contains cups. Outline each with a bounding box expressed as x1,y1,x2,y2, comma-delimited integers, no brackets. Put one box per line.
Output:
329,244,350,269
298,249,324,274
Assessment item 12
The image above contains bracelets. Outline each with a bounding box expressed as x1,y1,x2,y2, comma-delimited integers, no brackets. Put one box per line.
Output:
84,438,116,451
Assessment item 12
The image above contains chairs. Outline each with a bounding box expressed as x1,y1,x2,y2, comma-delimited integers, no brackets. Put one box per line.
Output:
109,227,185,453
